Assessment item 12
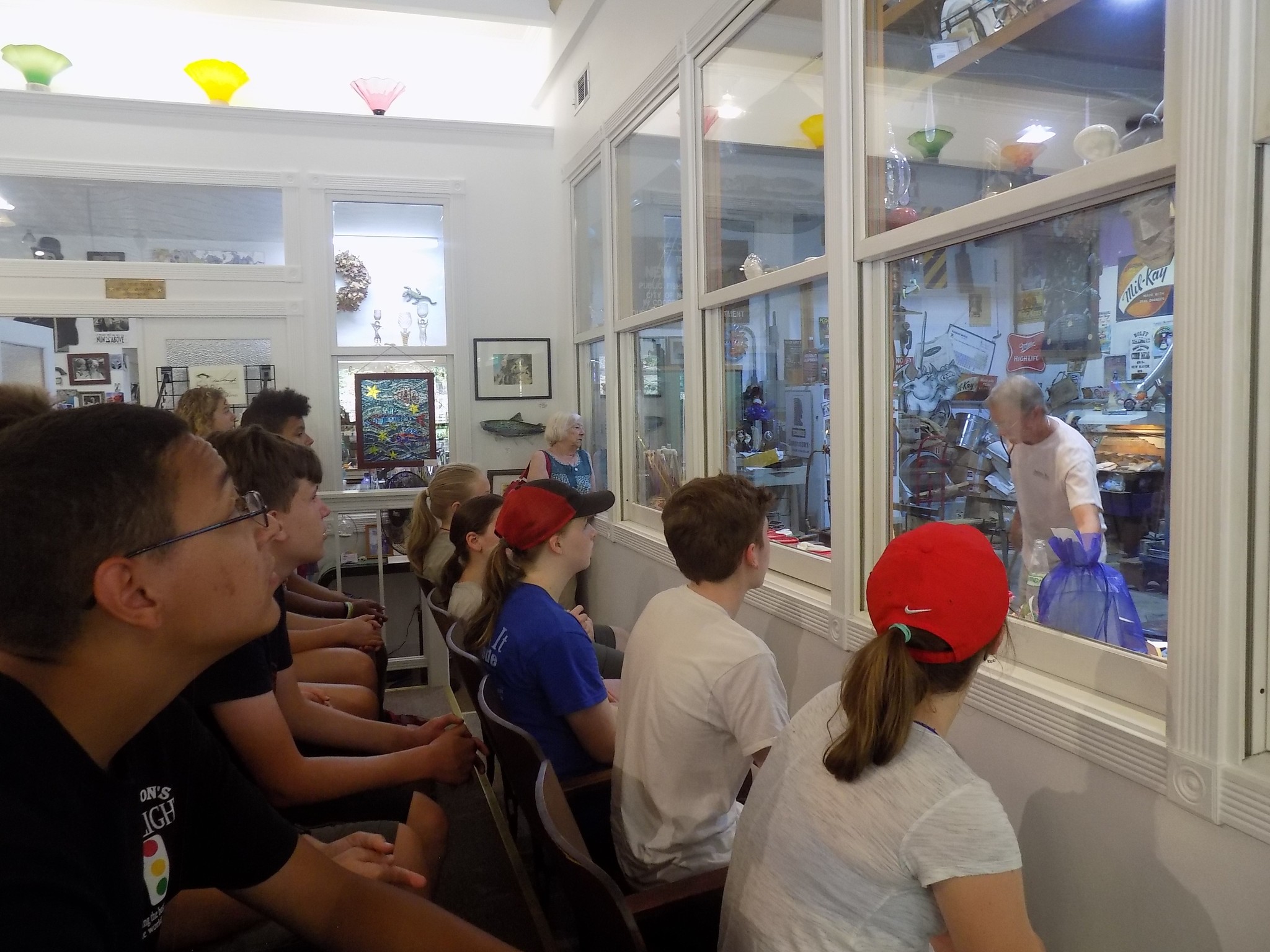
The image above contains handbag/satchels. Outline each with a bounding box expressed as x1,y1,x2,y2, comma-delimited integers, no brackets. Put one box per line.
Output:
503,450,551,499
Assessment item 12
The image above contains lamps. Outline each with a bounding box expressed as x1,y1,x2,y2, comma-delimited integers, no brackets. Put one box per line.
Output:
22,230,45,256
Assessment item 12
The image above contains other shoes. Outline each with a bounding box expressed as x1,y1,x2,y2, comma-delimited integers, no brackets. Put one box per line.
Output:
387,710,429,726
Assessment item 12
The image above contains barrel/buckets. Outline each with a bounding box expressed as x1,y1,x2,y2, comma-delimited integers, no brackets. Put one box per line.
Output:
955,412,990,453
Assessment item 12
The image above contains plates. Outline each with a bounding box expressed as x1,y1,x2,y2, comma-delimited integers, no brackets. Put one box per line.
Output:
939,0,1002,51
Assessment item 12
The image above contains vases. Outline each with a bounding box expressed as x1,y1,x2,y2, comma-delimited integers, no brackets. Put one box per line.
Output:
488,469,526,496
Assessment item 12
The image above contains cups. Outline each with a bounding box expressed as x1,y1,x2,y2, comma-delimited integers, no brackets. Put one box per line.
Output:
343,480,347,491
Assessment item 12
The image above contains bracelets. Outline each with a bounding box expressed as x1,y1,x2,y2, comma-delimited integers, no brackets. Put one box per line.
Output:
345,602,354,619
341,601,348,618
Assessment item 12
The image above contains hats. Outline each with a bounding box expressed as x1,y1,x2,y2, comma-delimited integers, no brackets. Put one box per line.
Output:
494,479,615,550
30,237,63,259
867,522,1008,661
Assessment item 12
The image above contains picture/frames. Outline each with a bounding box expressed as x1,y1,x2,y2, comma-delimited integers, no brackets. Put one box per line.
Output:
67,353,111,385
474,338,552,400
77,391,106,408
354,373,438,468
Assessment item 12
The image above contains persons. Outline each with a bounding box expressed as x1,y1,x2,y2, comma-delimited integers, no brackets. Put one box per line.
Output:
0,380,517,952
720,522,1043,952
440,493,629,679
609,475,791,889
406,462,490,581
986,374,1107,620
465,476,620,778
527,411,596,615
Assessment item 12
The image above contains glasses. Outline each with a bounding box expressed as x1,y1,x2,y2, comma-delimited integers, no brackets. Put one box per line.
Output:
989,414,1029,433
84,491,269,609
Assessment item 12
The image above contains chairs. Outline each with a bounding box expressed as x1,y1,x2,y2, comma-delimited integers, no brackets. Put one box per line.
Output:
414,573,727,952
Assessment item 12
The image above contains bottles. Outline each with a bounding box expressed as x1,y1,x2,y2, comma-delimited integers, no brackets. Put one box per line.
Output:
802,340,818,383
361,473,370,489
821,368,827,381
886,122,911,205
1024,539,1050,621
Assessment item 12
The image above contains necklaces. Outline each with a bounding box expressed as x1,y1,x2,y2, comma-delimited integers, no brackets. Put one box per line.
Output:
565,454,578,471
440,527,450,531
913,720,939,736
1046,416,1050,438
